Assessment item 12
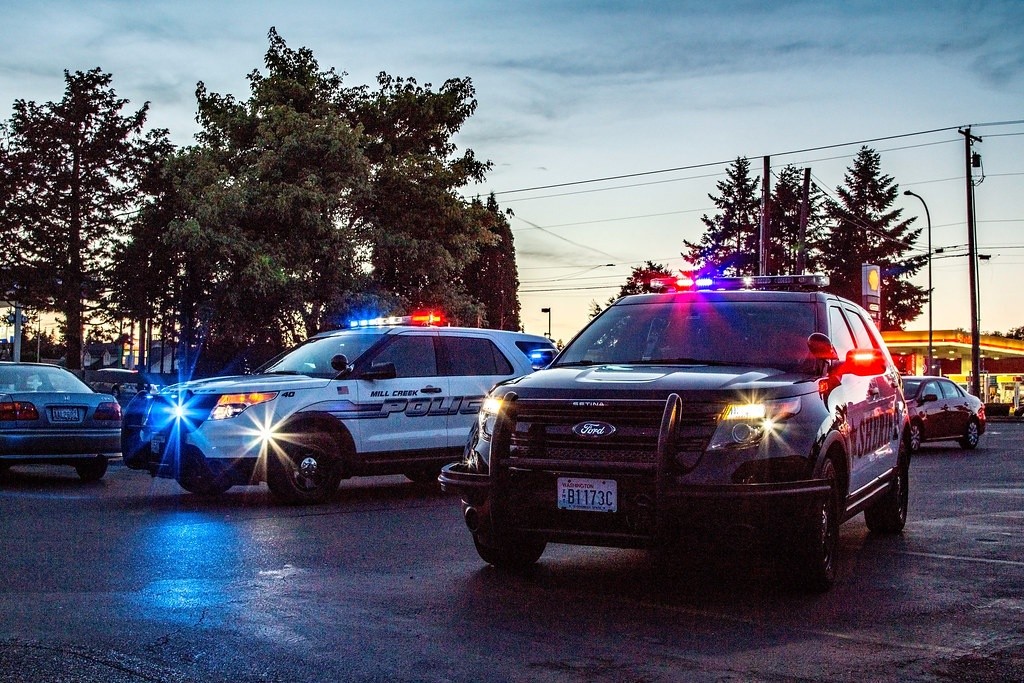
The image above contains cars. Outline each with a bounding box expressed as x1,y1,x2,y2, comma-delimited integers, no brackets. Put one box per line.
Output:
0,359,123,483
901,375,988,456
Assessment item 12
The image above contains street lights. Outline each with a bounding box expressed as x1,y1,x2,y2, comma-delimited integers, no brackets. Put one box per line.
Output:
902,189,933,376
541,307,551,340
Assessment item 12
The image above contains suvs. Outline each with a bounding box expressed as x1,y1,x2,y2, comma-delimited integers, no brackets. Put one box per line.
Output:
137,316,558,508
437,274,912,598
